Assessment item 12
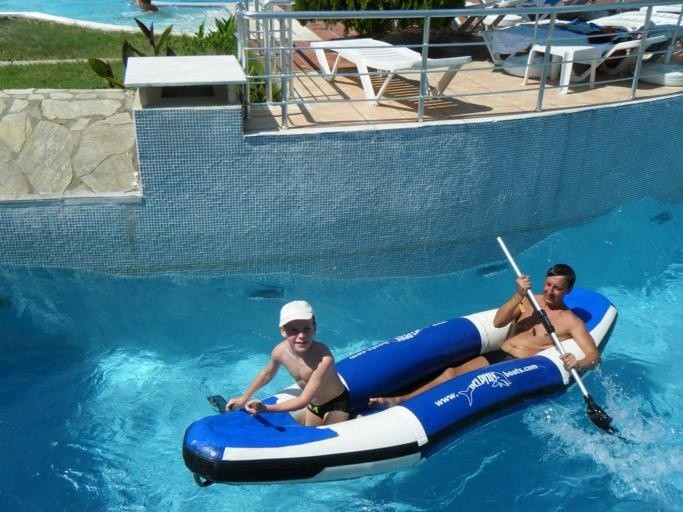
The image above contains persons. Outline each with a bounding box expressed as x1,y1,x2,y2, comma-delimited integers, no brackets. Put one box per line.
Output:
367,264,601,409
225,300,352,428
134,0,158,14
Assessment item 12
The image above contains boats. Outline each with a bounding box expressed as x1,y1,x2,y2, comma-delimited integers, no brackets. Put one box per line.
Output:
183,286,619,485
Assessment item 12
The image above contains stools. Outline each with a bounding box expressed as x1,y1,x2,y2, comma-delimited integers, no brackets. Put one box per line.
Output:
523,43,597,96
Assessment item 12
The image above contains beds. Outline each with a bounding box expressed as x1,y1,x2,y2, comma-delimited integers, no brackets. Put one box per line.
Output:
480,4,683,83
309,37,472,105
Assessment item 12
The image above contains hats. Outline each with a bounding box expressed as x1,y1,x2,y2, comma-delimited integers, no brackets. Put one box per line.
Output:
279,300,315,327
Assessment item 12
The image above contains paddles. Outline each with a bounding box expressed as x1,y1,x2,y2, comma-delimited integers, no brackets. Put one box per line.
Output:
496,235,634,445
206,395,264,413
